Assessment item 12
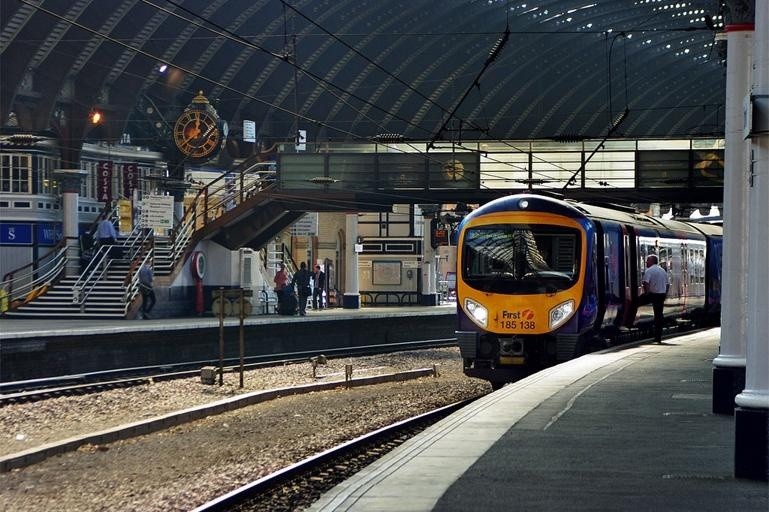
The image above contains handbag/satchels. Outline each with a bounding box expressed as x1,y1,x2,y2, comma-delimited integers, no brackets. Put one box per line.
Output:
140,284,151,295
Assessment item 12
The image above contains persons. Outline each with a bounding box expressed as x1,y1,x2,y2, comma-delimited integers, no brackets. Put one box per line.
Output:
220,184,236,213
310,265,325,310
273,263,289,311
614,254,671,347
291,261,313,316
97,215,117,259
136,259,158,320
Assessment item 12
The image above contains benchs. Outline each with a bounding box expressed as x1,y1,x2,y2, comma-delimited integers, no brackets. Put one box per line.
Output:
243,283,278,313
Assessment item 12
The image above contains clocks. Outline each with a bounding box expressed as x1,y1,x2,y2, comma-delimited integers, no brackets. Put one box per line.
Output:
173,90,229,165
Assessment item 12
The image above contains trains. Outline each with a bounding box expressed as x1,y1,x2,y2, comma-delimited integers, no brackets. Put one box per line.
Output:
452,192,723,380
